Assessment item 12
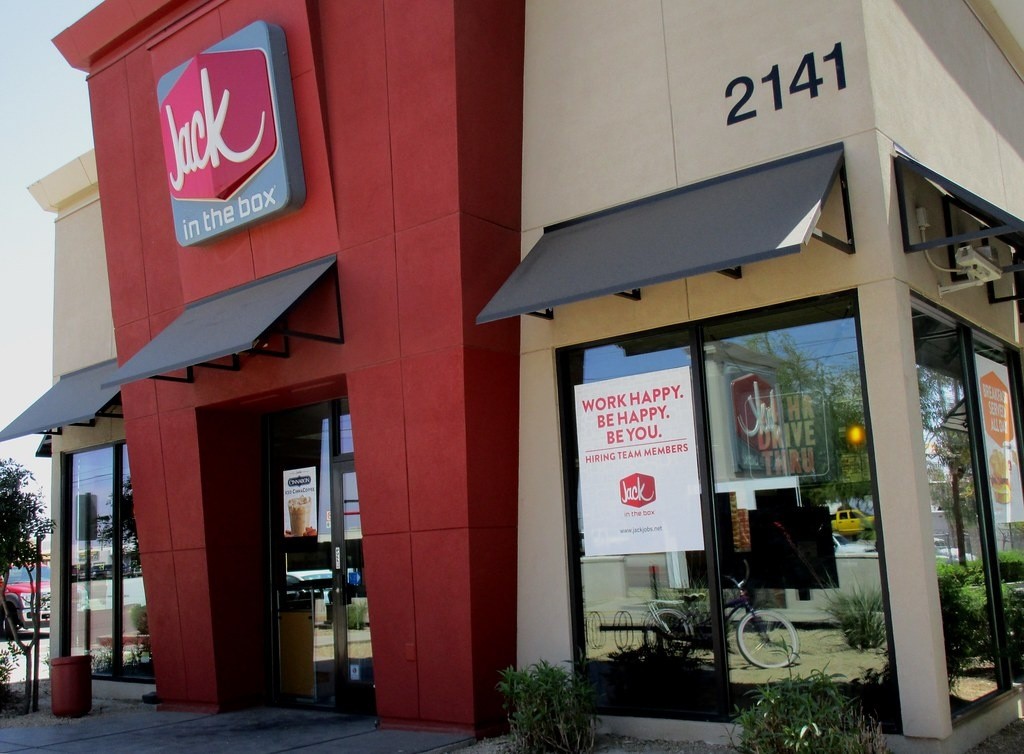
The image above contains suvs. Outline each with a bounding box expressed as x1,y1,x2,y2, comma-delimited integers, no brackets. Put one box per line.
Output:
1,565,50,641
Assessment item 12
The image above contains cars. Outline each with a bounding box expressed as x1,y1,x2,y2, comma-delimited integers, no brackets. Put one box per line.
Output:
831,534,877,553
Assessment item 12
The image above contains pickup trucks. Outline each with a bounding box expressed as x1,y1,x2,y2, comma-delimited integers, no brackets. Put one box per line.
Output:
830,506,875,532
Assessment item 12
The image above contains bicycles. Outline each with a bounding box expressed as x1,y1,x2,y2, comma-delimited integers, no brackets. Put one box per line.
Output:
641,556,799,668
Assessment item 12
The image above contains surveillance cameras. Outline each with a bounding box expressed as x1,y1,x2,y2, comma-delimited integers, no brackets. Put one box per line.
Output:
954,246,1003,282
